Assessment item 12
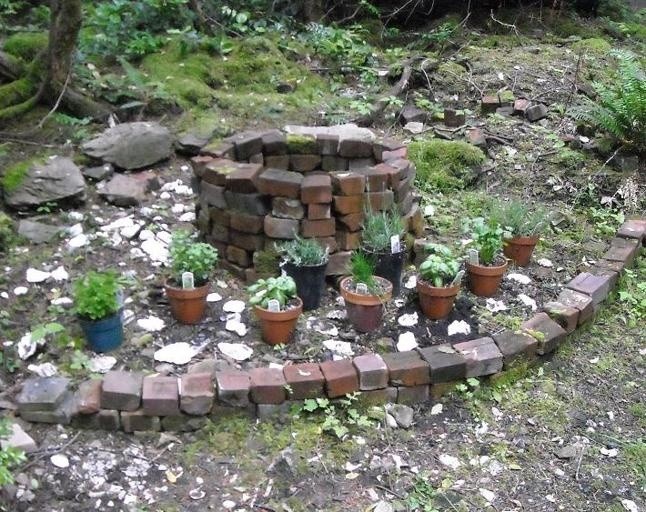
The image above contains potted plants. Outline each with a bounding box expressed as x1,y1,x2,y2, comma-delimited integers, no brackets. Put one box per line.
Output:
463,212,509,297
502,198,537,269
272,227,329,310
357,204,408,299
244,273,303,347
74,267,125,355
416,242,460,322
338,246,395,335
160,226,218,322
